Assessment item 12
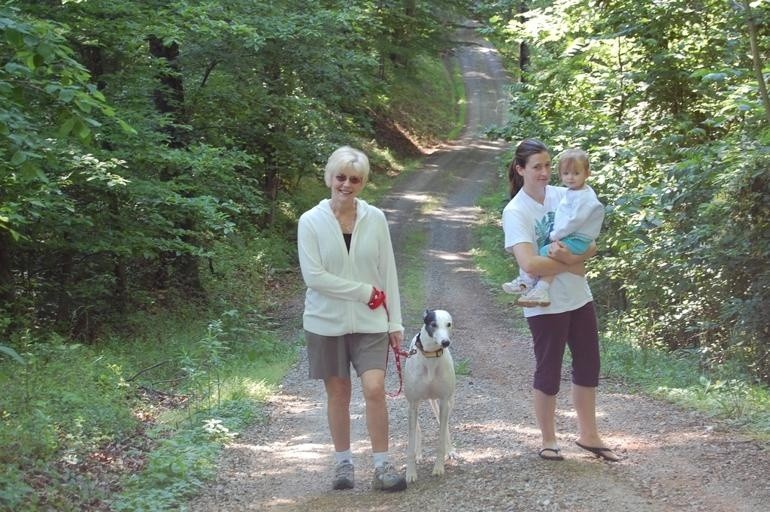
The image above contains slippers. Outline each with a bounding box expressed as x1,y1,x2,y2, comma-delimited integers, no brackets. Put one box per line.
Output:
576,440,619,461
538,446,563,460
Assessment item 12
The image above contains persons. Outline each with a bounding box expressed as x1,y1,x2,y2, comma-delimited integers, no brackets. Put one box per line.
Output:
502,137,620,462
502,149,605,307
297,146,406,492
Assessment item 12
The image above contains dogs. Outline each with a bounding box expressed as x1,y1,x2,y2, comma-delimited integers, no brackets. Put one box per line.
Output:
403,309,458,482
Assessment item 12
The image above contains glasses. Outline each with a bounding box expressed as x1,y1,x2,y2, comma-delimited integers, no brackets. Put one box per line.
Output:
334,173,361,184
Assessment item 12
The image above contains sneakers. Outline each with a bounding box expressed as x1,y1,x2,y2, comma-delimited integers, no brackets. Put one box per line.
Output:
373,463,406,490
333,464,354,490
